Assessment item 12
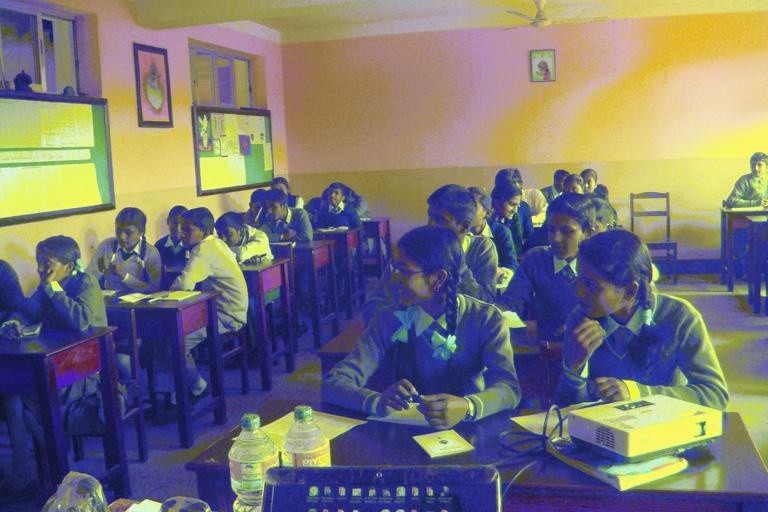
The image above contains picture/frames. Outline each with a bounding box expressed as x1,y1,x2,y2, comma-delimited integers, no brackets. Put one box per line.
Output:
529,48,557,83
132,42,173,130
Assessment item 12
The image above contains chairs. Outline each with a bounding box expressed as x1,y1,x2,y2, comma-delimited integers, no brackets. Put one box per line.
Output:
627,192,678,285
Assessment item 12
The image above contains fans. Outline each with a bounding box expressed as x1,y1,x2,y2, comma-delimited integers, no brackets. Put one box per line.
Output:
499,0,609,32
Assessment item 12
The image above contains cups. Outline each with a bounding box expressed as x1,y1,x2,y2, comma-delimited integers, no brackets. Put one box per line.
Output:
65,84,74,95
4,79,16,91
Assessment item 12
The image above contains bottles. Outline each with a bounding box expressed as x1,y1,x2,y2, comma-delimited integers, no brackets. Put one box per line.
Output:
282,404,330,467
15,68,32,91
228,412,281,512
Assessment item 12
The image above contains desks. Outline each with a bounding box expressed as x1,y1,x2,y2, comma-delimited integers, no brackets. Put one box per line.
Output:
106,215,392,461
315,248,569,411
720,199,768,315
185,394,768,511
2,325,131,512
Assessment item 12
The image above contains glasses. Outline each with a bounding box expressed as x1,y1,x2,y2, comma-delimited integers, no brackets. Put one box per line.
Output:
388,263,423,279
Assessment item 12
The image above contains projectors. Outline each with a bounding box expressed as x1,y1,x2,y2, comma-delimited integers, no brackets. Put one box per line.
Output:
567,394,725,463
